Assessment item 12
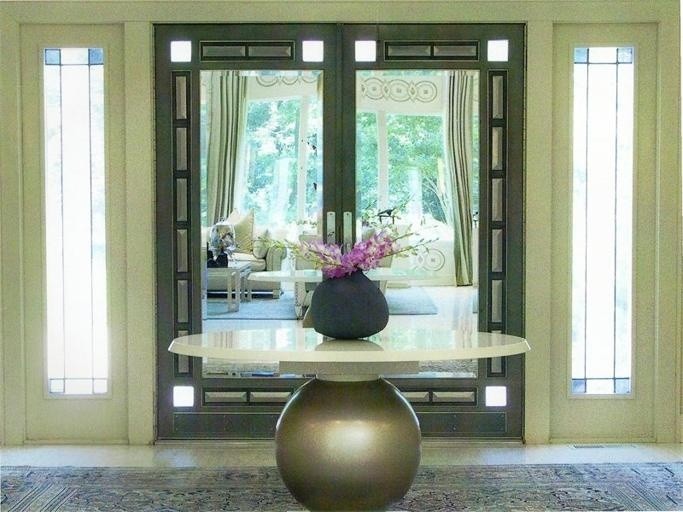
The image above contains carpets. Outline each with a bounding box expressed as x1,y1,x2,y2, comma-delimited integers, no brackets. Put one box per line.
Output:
0,460,683,512
203,283,439,320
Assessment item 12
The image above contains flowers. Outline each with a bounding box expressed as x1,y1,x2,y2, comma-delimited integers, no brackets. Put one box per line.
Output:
254,221,438,280
291,192,415,229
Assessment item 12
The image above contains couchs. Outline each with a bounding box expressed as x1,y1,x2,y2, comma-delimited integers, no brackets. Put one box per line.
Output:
206,227,287,299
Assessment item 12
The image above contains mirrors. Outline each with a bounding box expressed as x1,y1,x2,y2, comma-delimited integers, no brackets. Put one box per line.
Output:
342,22,524,440
154,23,341,444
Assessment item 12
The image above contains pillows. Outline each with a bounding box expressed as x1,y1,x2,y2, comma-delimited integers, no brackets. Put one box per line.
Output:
232,206,256,253
252,227,273,260
388,239,456,287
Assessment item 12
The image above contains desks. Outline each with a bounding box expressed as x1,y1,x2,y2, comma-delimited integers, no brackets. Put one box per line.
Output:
247,269,409,328
205,260,252,310
168,325,530,512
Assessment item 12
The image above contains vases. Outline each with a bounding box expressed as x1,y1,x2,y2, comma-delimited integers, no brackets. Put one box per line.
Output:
310,267,390,340
